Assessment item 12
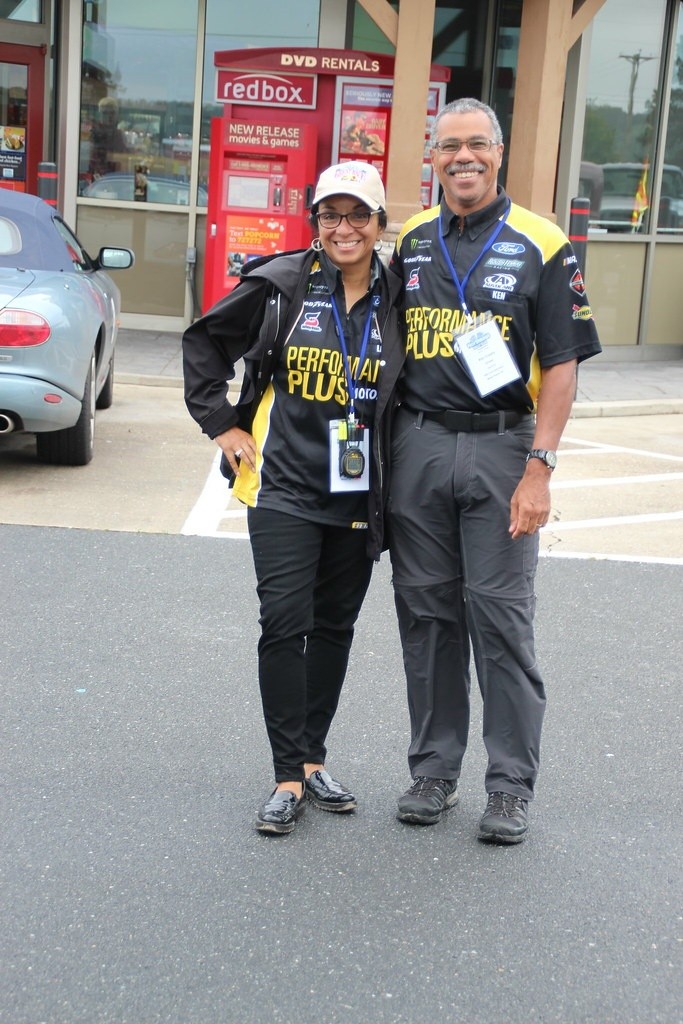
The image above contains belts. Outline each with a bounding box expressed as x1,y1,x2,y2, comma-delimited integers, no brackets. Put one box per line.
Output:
401,403,519,433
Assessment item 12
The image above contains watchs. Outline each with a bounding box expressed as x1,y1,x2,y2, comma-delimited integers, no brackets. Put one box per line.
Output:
526,450,557,469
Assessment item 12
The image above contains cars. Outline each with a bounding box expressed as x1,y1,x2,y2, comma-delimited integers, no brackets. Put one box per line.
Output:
0,188,122,467
80,173,211,210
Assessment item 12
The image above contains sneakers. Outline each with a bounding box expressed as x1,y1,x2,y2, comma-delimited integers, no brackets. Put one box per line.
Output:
396,776,459,825
255,780,306,833
477,791,528,842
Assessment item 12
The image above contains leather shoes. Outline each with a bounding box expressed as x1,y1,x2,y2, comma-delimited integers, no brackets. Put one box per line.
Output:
304,769,357,812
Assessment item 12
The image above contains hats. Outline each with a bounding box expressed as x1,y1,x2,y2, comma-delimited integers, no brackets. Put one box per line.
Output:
313,160,385,211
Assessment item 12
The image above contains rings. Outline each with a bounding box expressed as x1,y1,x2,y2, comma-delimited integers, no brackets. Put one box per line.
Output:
537,524,543,527
235,449,243,457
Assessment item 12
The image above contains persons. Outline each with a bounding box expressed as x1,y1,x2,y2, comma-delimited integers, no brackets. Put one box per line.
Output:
181,159,407,834
389,98,603,835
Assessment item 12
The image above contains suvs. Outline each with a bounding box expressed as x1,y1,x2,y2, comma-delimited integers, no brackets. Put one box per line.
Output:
592,160,683,232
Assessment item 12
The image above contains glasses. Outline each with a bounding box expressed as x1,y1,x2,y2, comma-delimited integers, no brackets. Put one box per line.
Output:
434,137,498,153
316,209,381,229
